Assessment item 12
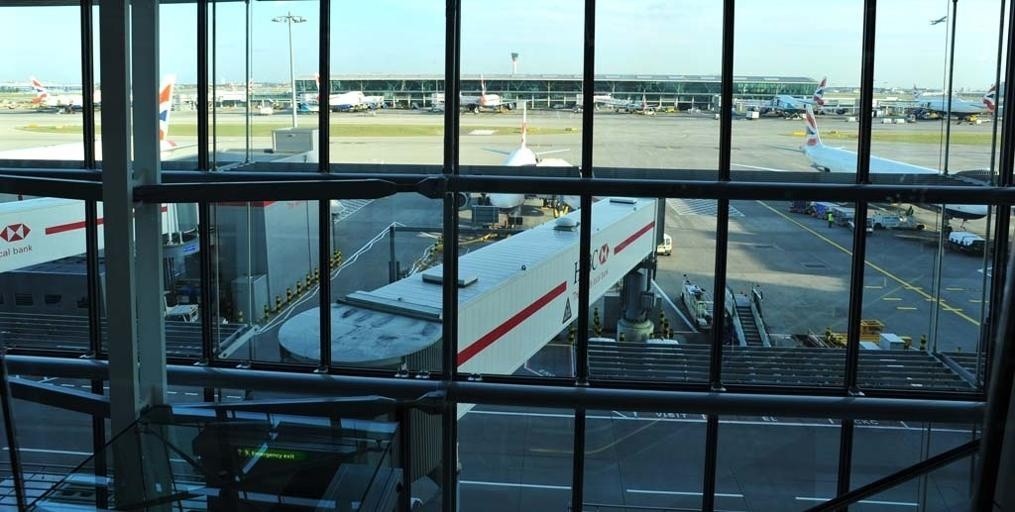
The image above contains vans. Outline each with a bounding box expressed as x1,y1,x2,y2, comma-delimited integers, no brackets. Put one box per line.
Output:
656,234,673,255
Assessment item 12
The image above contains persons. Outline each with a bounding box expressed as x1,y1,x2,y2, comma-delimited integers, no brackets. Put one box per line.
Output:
907,205,914,216
827,211,835,229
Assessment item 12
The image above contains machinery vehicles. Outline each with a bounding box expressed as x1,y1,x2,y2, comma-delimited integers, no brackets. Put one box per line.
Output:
789,200,924,235
948,232,985,257
679,272,714,332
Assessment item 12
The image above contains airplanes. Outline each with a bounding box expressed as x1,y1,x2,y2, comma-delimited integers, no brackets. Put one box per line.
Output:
798,104,989,229
879,82,988,123
310,74,385,111
978,85,995,115
734,76,860,119
455,100,571,213
27,75,102,115
617,91,666,117
460,73,533,113
0,73,177,164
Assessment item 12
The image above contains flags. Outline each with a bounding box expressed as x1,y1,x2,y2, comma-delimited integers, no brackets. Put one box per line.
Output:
158,82,170,141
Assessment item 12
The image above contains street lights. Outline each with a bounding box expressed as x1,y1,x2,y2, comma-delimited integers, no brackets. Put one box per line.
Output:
273,9,306,128
510,52,519,73
